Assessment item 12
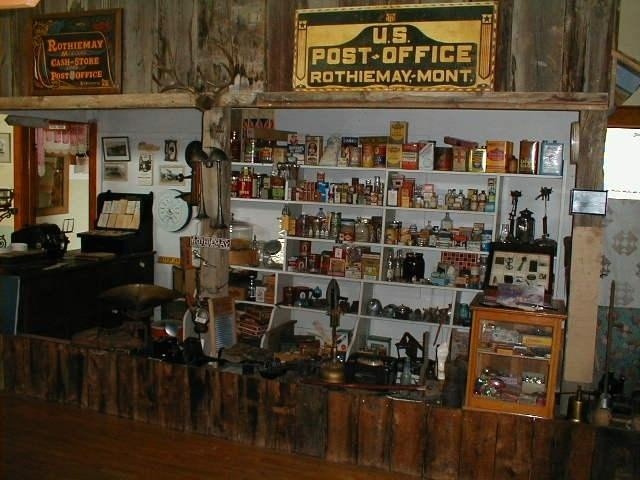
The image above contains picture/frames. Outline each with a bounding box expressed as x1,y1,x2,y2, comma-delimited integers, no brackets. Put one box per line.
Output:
100,135,131,162
0,132,12,164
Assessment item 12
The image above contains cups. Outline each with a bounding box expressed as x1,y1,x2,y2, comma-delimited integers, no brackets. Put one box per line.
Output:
256,287,264,303
501,223,509,238
283,286,293,306
509,158,518,173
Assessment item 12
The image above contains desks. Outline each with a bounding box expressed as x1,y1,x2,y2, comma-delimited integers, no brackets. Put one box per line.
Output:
0,243,64,339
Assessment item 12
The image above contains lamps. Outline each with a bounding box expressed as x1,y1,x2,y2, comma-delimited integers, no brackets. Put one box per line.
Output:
0,187,19,224
182,139,231,230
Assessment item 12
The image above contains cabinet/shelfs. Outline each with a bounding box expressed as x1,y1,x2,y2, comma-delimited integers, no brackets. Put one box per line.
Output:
76,189,156,286
458,291,567,424
228,155,567,382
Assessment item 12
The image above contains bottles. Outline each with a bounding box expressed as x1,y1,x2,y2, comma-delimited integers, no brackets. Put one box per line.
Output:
409,206,492,251
413,178,496,212
357,222,368,242
387,249,488,286
248,234,260,265
366,175,385,206
301,207,326,236
459,303,469,320
248,275,256,298
230,129,273,162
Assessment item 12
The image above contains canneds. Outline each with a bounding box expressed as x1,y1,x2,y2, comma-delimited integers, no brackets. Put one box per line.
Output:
291,171,325,274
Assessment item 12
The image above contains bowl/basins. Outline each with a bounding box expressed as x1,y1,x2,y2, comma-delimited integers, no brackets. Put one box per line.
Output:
151,319,183,341
